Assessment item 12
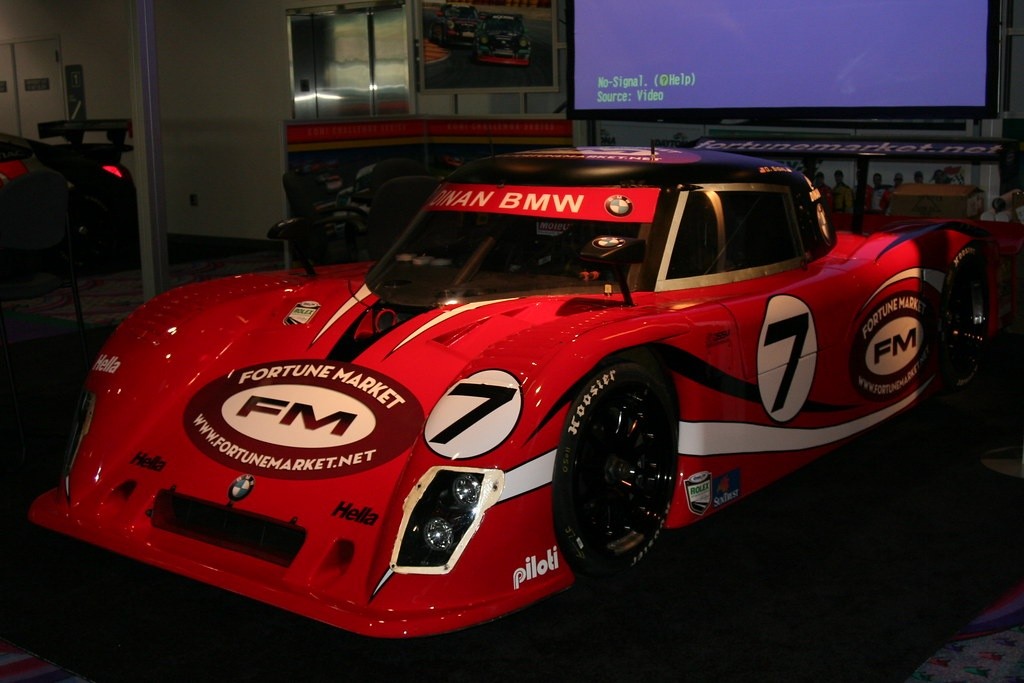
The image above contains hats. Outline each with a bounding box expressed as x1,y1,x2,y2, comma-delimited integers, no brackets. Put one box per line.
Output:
914,171,922,177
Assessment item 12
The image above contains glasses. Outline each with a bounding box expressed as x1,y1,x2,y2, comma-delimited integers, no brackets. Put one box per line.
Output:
894,178,902,181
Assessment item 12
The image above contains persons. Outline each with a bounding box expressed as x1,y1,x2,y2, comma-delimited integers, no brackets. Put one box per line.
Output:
805,170,944,214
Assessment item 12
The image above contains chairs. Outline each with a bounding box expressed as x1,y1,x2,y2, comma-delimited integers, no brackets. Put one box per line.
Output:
354,158,441,192
0,167,92,464
365,174,456,261
283,171,369,263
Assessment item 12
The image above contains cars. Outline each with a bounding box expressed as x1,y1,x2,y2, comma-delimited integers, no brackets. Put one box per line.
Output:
0,134,137,287
429,1,533,68
23,145,1024,642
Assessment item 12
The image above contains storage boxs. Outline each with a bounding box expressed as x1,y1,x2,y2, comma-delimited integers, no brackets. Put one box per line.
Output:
891,182,985,219
